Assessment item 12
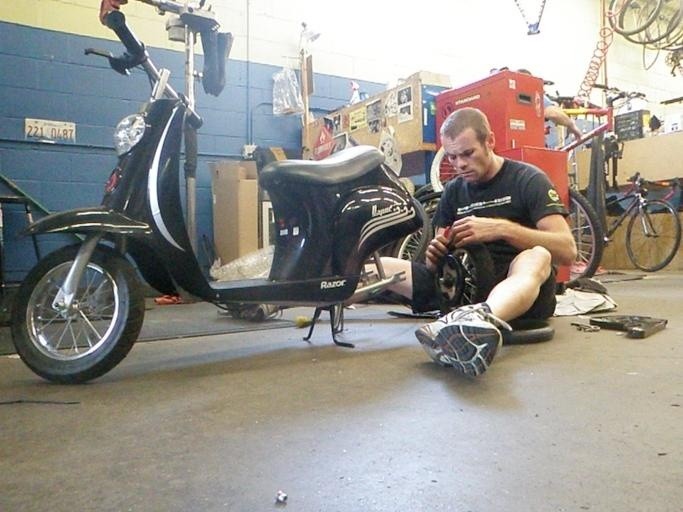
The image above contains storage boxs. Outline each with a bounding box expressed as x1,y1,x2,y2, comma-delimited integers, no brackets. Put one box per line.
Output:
433,70,569,221
209,159,258,265
301,73,453,194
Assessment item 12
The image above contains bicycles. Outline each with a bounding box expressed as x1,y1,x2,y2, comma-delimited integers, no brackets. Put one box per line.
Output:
536,74,683,284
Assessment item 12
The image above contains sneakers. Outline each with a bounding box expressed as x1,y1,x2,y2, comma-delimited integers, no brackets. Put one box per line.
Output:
225,299,279,322
413,302,505,381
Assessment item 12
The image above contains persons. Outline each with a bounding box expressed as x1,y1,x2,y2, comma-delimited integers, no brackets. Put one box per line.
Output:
516,69,583,150
225,107,578,378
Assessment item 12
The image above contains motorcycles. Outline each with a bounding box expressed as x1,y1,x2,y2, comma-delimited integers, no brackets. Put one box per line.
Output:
9,0,420,384
